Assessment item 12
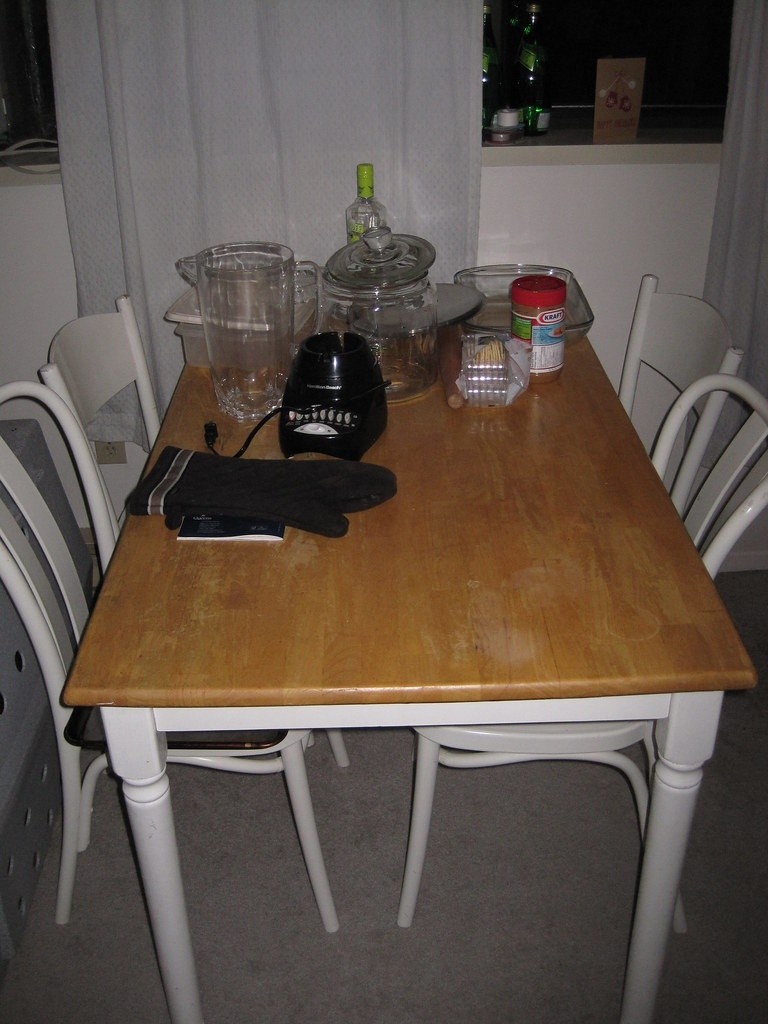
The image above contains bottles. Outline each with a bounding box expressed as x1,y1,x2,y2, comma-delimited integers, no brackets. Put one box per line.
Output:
515,4,551,135
345,163,386,243
322,226,439,406
482,5,501,141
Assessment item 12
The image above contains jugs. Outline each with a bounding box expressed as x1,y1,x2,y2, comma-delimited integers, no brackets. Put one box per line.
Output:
177,242,321,423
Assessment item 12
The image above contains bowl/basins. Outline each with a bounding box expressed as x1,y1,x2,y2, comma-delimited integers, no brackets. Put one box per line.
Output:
454,263,595,350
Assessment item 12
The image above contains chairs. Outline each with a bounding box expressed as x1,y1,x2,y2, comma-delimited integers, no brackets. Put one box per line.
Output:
0,380,353,930
616,274,746,517
395,374,768,933
38,293,163,541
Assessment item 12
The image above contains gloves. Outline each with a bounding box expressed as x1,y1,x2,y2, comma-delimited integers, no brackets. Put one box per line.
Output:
125,446,397,538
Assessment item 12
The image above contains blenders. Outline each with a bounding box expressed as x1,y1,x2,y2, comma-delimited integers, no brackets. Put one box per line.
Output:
279,332,390,463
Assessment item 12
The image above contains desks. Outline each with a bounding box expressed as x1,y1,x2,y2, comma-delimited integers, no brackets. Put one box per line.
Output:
57,301,758,1024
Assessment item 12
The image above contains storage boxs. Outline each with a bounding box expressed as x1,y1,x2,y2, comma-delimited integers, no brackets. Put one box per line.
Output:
174,274,319,369
454,264,595,352
